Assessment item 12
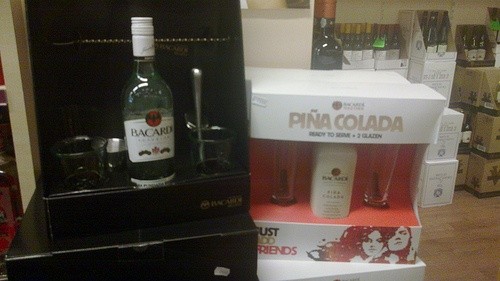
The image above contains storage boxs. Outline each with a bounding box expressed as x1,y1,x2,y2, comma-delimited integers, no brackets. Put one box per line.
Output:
242,7,500,281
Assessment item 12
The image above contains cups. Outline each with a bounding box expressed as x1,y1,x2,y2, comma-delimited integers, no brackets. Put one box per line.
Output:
49,135,107,191
189,125,236,174
271,136,299,205
363,144,399,207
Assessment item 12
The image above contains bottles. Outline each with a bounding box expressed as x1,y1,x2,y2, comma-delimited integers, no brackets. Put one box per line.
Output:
420,10,451,52
459,112,472,147
464,25,486,61
311,0,401,70
120,17,176,184
